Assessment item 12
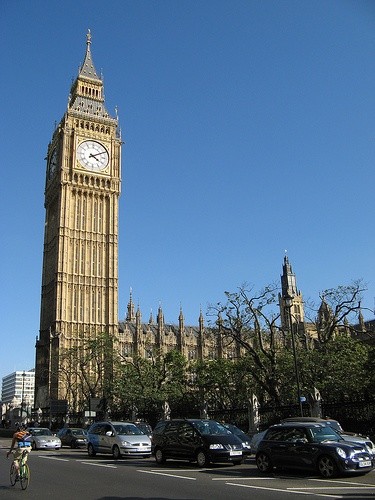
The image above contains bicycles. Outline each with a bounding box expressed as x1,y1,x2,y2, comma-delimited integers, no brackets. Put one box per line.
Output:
6,450,31,490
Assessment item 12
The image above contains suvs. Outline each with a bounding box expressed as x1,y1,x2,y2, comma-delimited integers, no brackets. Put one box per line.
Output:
254,422,375,479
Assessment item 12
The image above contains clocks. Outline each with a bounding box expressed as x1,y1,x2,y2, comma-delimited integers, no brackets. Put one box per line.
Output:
49,148,57,179
77,140,109,171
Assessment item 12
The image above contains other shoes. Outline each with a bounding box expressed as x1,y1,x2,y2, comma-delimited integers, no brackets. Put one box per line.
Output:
15,474,21,477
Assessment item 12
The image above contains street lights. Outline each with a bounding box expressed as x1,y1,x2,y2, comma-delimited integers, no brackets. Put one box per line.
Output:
47,326,55,402
21,369,27,414
283,292,305,417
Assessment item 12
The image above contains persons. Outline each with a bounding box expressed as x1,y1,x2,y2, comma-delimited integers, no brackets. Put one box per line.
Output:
6,426,33,483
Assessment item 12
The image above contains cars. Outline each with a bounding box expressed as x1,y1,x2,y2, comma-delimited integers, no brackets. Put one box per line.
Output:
85,420,153,460
56,427,87,449
27,427,62,451
251,416,375,463
127,418,253,468
